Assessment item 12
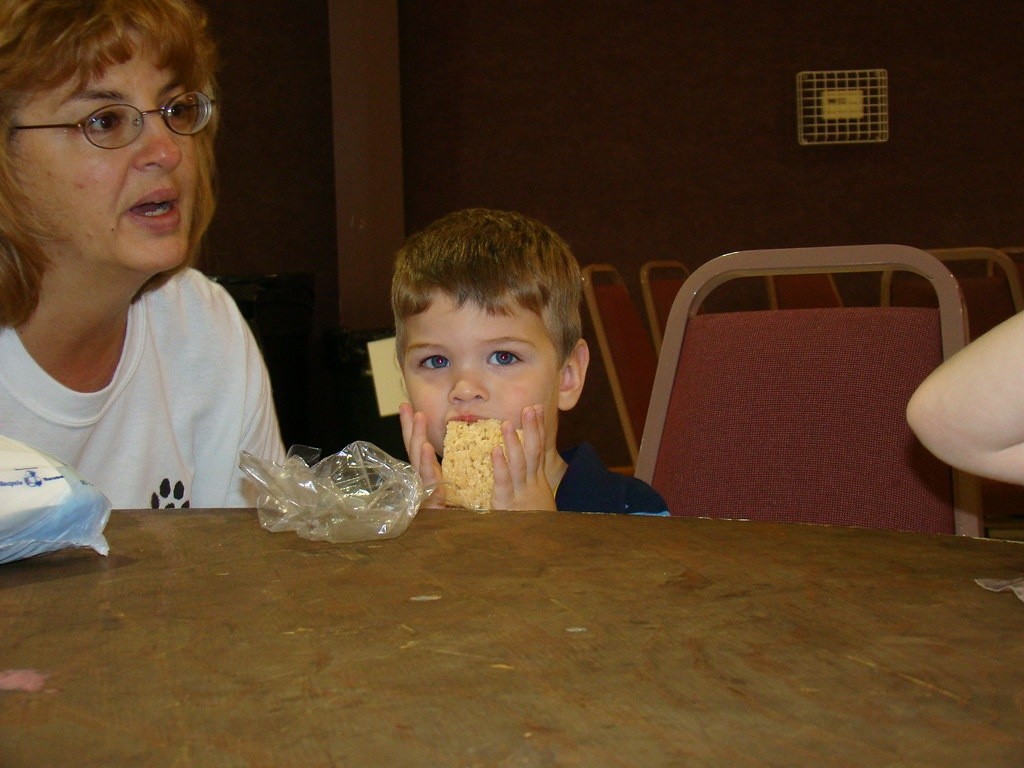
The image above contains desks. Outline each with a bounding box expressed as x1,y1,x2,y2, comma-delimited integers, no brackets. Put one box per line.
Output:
1,509,1024,768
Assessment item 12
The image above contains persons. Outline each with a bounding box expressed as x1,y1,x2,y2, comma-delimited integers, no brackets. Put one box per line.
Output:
0,0,297,511
904,305,1024,486
392,203,675,519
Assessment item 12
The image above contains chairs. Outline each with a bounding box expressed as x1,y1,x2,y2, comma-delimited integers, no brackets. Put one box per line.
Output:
586,246,1020,542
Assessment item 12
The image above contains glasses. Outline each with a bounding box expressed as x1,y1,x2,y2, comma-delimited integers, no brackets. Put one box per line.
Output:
14,90,217,150
